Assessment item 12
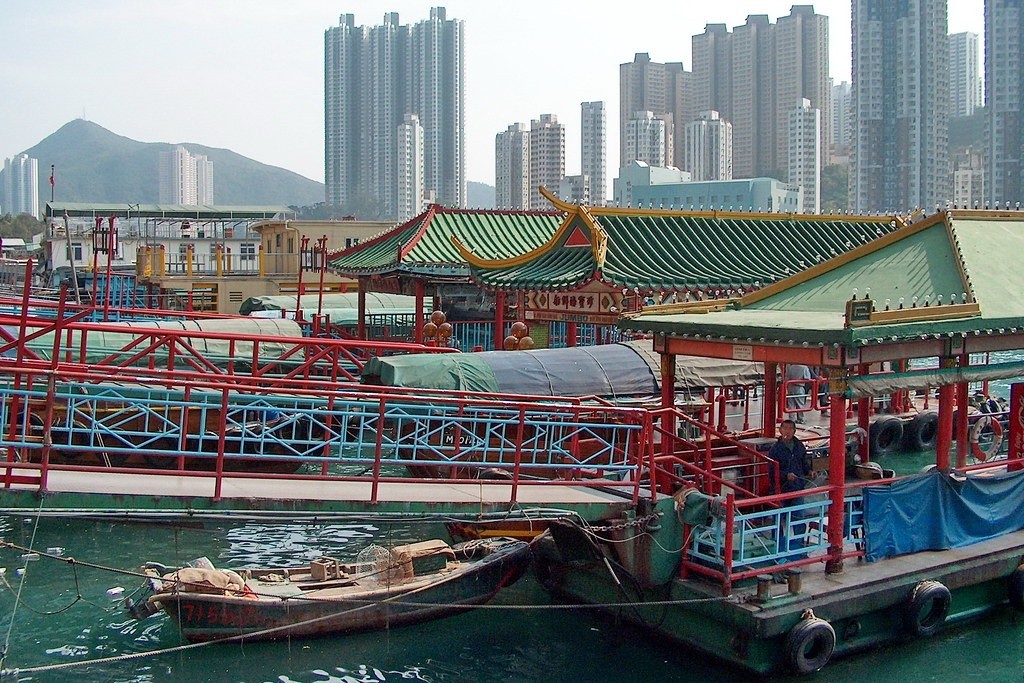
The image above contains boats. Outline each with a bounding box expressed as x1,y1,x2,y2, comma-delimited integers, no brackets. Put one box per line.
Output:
0,183,1024,673
126,535,535,644
444,518,550,547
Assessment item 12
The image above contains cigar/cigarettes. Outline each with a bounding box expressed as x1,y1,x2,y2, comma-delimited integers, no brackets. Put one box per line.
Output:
783,435,787,438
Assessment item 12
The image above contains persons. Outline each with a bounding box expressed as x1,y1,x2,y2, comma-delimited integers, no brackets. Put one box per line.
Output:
784,365,810,425
768,420,817,559
803,367,831,417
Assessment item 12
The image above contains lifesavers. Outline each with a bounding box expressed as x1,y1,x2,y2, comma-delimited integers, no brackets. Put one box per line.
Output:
970,417,1004,461
873,415,906,455
909,409,941,453
782,613,836,677
908,579,953,636
